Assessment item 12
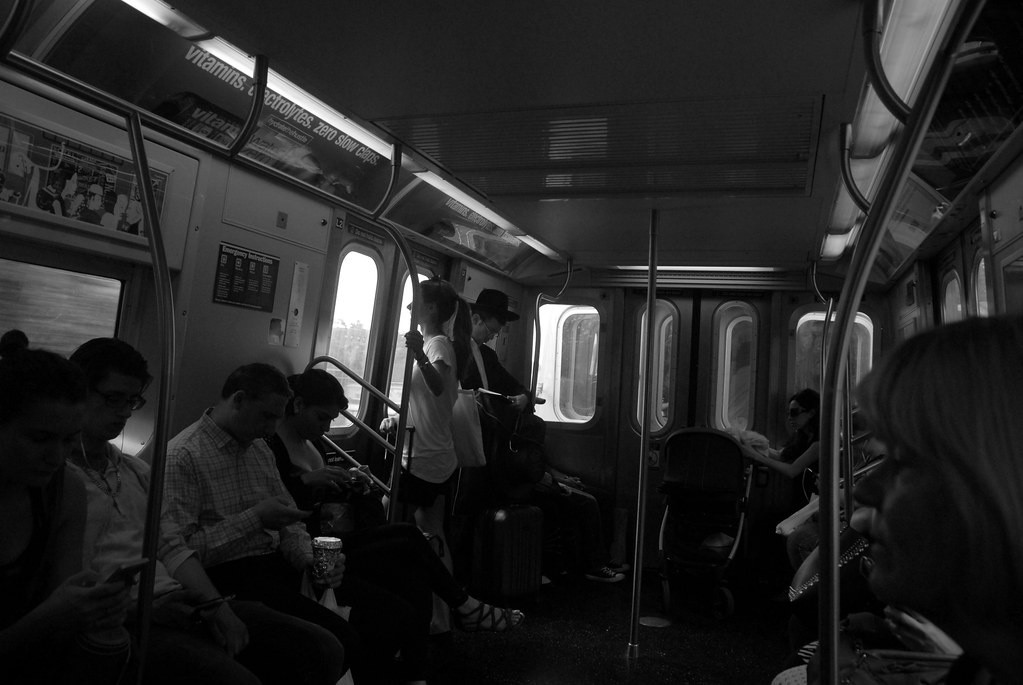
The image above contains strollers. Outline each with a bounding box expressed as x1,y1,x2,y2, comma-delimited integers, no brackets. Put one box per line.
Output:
640,424,771,625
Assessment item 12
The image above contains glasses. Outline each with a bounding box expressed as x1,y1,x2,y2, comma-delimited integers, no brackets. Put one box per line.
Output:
433,273,442,291
481,322,500,338
787,408,808,417
94,387,146,411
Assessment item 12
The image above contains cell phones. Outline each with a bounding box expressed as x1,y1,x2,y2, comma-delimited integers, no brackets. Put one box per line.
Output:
344,478,363,485
105,557,151,585
189,594,235,616
301,501,321,512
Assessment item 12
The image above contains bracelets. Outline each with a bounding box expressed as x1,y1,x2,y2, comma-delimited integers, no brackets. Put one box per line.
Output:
417,354,430,369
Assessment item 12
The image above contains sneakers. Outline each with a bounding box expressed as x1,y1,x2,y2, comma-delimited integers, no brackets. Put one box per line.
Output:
604,560,630,572
585,567,625,583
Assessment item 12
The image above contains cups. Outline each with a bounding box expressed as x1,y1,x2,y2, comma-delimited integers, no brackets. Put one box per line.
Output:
311,537,342,584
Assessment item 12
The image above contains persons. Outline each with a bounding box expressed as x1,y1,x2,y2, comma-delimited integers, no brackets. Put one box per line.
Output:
533,399,629,582
444,290,531,591
734,304,1023,685
0,328,530,685
379,277,475,642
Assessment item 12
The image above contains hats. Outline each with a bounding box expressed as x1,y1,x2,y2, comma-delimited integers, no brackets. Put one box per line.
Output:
468,289,520,322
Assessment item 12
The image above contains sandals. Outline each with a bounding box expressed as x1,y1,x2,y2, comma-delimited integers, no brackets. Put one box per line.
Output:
454,600,525,633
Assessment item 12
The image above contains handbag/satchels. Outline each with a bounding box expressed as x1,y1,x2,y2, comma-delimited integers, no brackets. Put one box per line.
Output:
482,391,546,485
806,612,961,685
